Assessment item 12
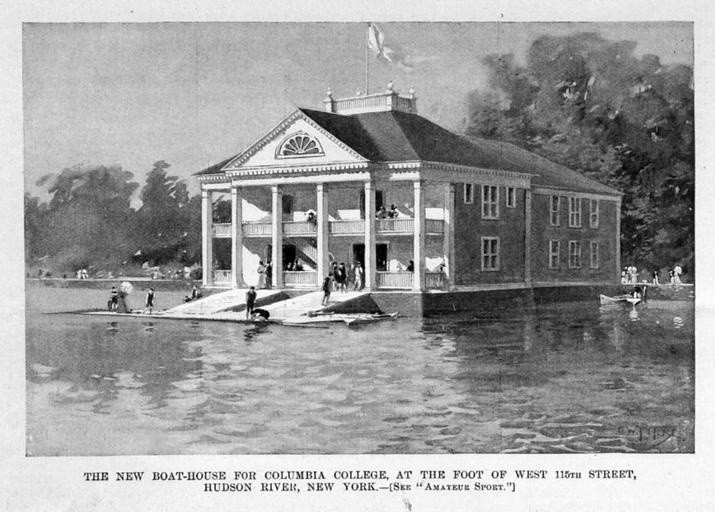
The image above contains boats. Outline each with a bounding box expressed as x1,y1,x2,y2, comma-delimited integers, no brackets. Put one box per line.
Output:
282,310,401,328
599,292,641,309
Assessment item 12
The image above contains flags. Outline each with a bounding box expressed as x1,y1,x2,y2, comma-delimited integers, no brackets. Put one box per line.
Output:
367,22,395,62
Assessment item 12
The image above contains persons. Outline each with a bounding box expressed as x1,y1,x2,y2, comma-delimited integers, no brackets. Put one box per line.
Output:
375,206,387,221
321,261,364,306
620,264,640,283
668,264,683,285
387,204,399,219
257,257,305,290
76,266,87,279
184,284,202,303
145,286,156,315
246,286,257,320
307,212,317,226
406,260,414,272
633,284,642,299
653,269,659,285
251,308,270,322
111,286,118,310
439,262,446,271
311,239,317,248
